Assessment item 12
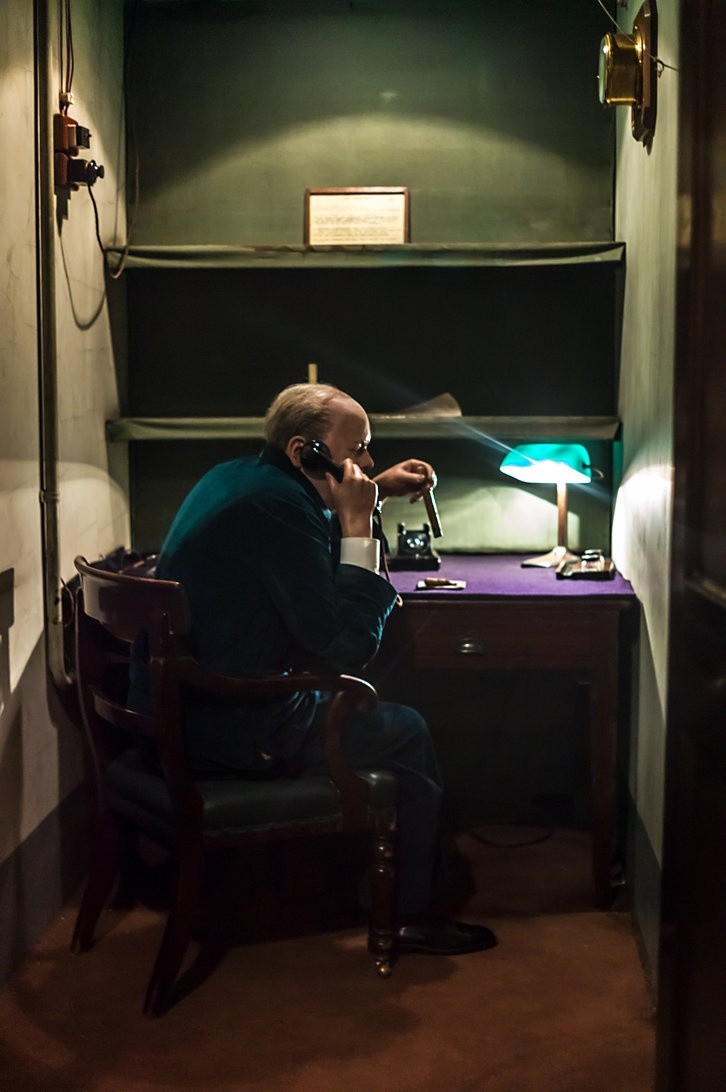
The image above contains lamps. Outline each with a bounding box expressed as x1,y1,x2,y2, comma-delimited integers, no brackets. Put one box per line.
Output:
500,440,604,570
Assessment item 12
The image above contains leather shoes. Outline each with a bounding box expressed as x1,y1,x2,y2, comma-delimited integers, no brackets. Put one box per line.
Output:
369,916,496,954
315,890,356,930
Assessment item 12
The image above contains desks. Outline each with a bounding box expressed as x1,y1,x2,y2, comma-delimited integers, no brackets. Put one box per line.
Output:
73,544,637,911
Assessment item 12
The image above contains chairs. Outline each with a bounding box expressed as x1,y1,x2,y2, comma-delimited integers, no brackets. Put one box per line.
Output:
58,551,411,1020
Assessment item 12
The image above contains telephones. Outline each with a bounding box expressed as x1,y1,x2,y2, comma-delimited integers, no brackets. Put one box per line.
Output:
300,440,382,516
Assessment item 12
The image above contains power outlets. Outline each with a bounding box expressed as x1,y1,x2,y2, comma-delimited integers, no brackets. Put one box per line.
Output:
55,110,79,157
52,152,80,192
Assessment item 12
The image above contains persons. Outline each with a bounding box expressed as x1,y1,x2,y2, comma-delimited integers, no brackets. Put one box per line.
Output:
127,382,498,956
